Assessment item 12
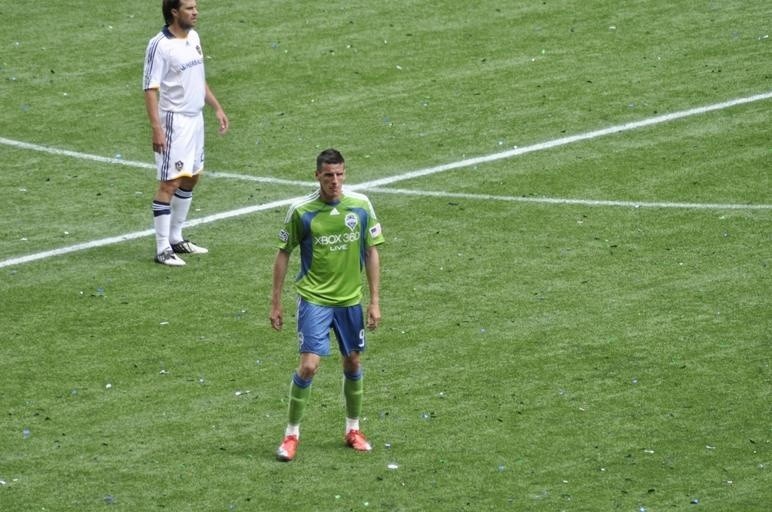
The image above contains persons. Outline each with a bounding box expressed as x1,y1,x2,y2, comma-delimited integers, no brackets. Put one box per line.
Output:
267,149,385,461
142,1,230,265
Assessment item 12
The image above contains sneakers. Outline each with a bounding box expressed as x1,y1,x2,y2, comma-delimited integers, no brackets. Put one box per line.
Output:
344,429,372,451
155,247,186,266
276,434,299,461
171,240,208,253
